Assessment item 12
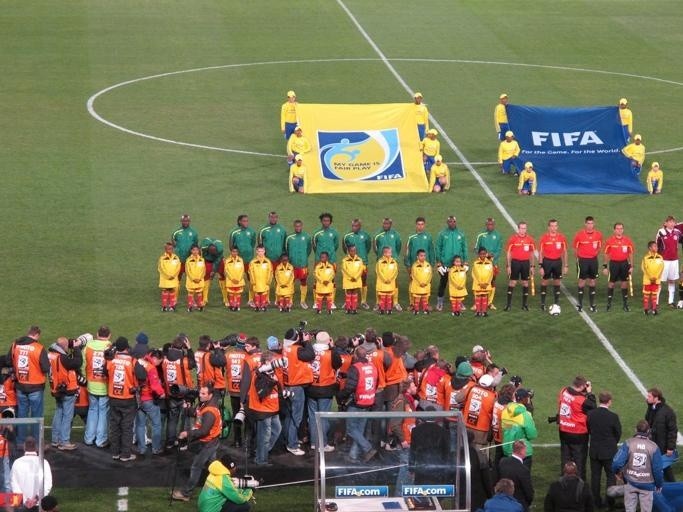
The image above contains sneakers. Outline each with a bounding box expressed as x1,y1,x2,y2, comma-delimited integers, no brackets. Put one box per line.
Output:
576,305,631,313
225,304,295,312
300,303,308,310
172,491,189,501
437,304,445,312
312,302,371,315
373,304,434,315
113,438,179,462
161,304,208,313
52,441,61,447
502,304,548,311
452,303,497,317
643,300,682,316
233,438,411,468
58,443,78,450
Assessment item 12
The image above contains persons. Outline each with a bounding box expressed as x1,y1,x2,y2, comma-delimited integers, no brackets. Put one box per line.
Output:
414,92,429,141
504,221,536,311
618,97,633,144
286,126,311,165
0,325,677,512
309,213,402,314
641,215,682,316
572,216,603,312
280,90,300,143
419,129,440,176
621,133,645,176
288,154,306,194
493,94,510,142
498,130,521,177
428,155,450,194
537,220,568,311
517,161,537,195
158,211,312,312
602,222,635,312
646,161,664,194
404,214,503,317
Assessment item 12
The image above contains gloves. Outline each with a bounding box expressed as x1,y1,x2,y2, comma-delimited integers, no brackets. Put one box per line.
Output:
437,266,447,276
463,265,469,272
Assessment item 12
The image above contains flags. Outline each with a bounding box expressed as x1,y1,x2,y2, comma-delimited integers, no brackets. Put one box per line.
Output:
504,104,648,194
288,104,432,192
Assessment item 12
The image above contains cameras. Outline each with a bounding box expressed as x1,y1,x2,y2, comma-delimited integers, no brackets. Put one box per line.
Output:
130,386,141,395
547,413,560,424
298,320,318,340
56,382,68,391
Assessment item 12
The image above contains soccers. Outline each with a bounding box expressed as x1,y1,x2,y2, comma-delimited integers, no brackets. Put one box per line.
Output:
549,304,561,317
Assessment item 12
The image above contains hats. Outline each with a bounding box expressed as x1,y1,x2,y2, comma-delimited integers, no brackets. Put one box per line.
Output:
295,126,302,131
434,155,443,161
266,328,331,350
525,161,533,168
414,93,423,98
499,94,507,99
295,154,302,161
634,134,641,140
428,129,438,135
619,98,627,105
515,390,532,398
136,333,148,343
505,131,513,137
456,344,494,388
652,162,659,168
221,455,237,470
287,91,296,97
